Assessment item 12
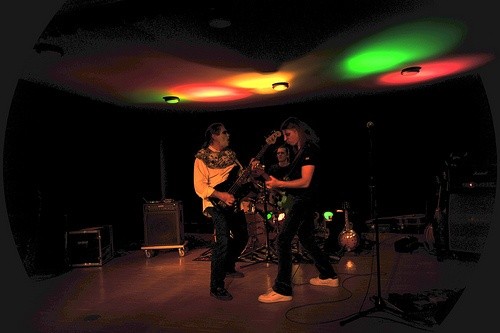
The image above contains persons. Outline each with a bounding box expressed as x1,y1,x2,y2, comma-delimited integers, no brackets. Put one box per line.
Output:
259,116,339,303
194,122,291,301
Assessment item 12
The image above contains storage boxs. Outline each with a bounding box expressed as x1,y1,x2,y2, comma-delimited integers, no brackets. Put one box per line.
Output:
64,225,114,267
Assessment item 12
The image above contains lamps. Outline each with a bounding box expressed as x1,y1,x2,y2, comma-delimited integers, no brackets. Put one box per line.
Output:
165,96,180,104
35,42,64,56
401,66,421,76
273,82,288,91
267,211,287,227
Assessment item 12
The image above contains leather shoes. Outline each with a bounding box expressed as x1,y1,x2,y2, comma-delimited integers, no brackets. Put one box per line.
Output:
225,270,244,277
210,287,232,300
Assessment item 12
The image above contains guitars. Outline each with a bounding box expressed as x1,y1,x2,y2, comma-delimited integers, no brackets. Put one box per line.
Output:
338,201,359,251
209,130,283,218
249,158,290,208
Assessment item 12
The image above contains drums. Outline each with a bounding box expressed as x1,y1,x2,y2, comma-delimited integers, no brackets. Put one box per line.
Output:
214,202,283,253
240,198,255,214
256,189,283,214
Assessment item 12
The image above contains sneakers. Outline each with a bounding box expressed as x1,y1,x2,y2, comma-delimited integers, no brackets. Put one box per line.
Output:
308,274,340,287
258,288,293,303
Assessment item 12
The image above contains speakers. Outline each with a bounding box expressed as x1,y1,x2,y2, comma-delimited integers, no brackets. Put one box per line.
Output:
142,212,184,246
23,238,65,274
449,193,496,254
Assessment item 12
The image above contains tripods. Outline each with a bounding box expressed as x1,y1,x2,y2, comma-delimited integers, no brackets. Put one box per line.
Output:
339,183,425,326
237,178,278,269
